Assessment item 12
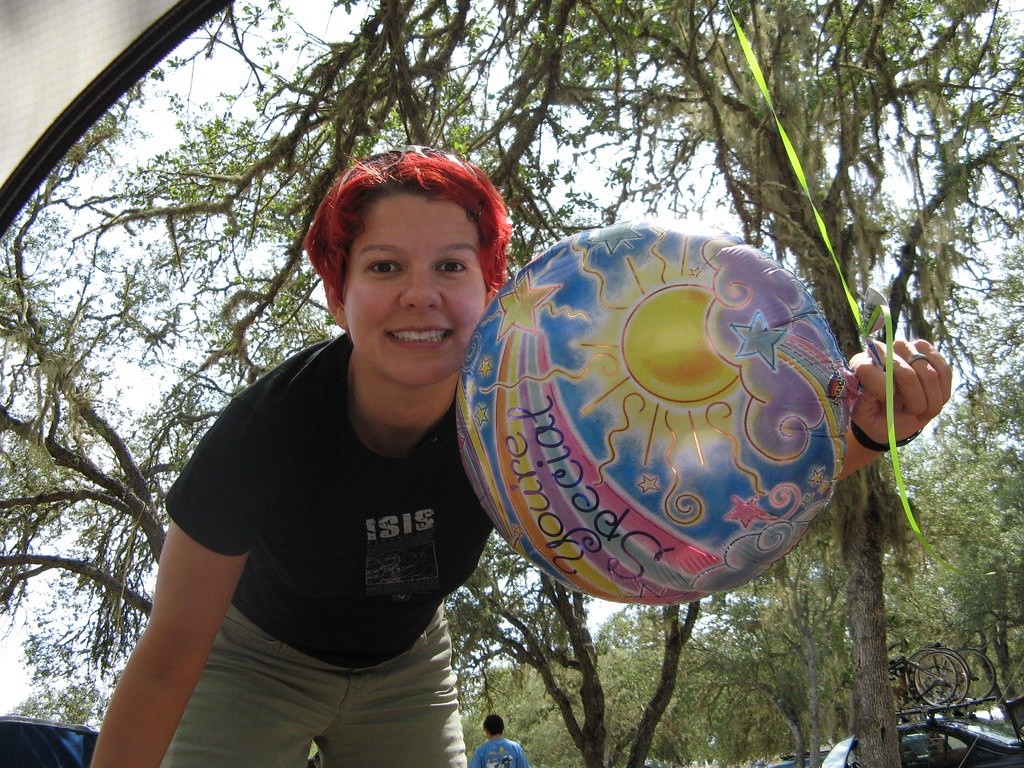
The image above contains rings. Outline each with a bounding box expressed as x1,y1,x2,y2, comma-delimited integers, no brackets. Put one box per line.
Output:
906,354,931,366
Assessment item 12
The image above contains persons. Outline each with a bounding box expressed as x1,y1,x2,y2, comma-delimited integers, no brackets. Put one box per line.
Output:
93,146,953,768
470,713,532,768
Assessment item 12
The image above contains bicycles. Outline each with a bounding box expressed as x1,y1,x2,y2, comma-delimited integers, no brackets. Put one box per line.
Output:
887,637,997,707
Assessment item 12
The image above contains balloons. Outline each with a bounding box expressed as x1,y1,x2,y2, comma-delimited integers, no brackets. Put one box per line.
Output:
456,218,863,606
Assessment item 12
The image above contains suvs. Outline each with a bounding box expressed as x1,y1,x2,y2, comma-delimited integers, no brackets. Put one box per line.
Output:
818,694,1024,768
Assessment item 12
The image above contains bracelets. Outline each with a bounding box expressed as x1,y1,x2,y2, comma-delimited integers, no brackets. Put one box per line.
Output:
850,417,924,451
